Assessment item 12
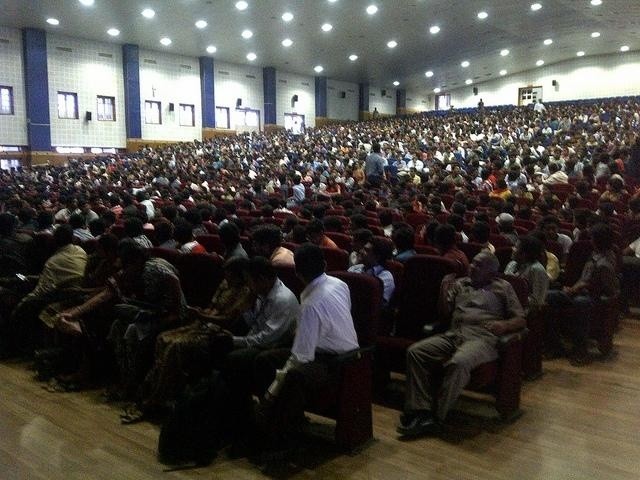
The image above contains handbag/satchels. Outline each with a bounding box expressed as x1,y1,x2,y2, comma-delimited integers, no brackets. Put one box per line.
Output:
160,396,215,464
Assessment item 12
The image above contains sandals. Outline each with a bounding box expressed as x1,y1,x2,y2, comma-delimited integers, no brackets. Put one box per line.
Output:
120,400,144,423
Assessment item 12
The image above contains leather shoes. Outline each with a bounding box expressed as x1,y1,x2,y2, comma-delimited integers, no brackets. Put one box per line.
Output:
397,411,443,436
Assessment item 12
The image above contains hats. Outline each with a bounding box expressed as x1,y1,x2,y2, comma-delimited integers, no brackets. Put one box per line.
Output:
494,212,515,227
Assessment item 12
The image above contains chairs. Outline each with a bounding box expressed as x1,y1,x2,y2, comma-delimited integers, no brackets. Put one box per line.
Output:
1,176,640,449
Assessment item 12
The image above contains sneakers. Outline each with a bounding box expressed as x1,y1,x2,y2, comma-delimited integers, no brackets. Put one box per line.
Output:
30,348,82,394
